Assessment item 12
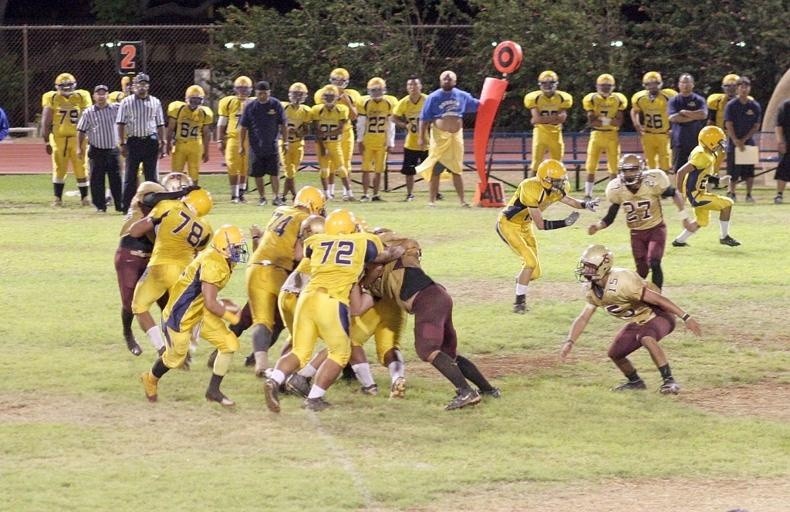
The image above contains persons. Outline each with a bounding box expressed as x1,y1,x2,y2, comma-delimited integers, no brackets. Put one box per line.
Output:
496,159,599,314
115,172,500,412
559,244,702,395
41,68,479,215
587,154,690,293
671,126,742,247
0,109,9,141
524,70,790,204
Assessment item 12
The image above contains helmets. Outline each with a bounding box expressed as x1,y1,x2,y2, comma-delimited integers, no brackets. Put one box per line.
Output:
235,68,385,107
574,243,613,282
596,72,616,96
539,70,558,93
296,186,363,239
698,125,729,155
536,159,569,190
161,171,250,264
722,71,741,89
54,72,79,98
643,71,662,92
618,154,646,187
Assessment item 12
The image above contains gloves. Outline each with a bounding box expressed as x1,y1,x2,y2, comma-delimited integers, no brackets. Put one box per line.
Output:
585,197,600,210
566,212,580,227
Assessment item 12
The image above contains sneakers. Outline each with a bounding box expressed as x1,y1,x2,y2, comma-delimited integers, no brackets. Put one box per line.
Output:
125,337,233,406
445,388,499,410
660,378,678,393
245,353,406,413
726,192,785,204
672,238,688,247
720,234,741,247
514,293,527,313
613,380,646,391
259,196,296,207
363,192,472,209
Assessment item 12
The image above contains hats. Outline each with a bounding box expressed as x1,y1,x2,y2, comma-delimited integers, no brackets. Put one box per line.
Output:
185,85,207,101
94,83,109,92
132,72,149,84
